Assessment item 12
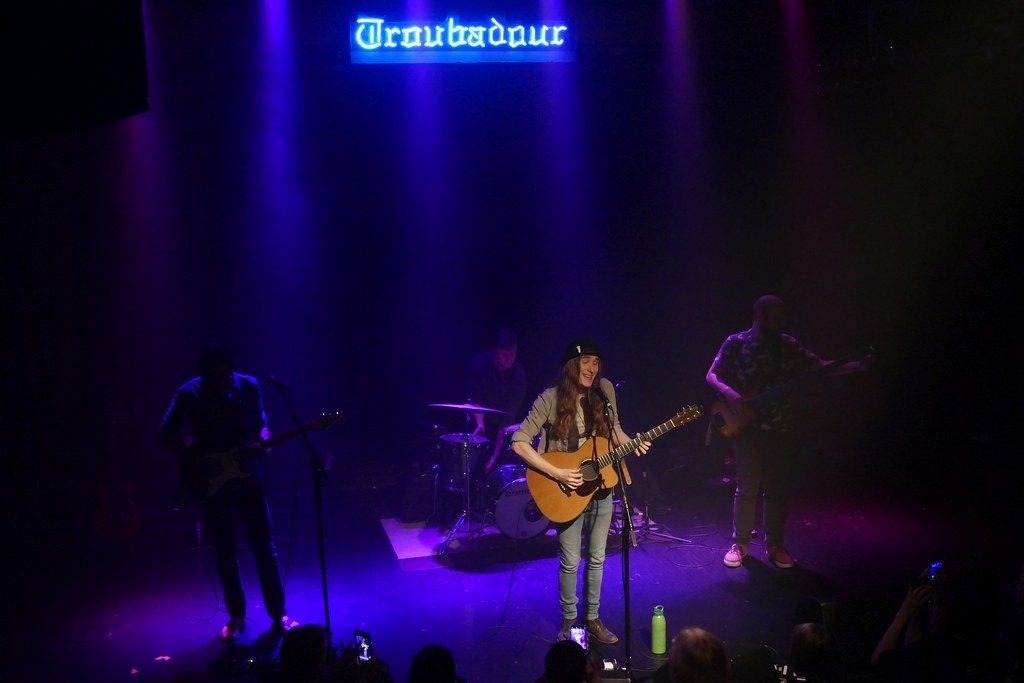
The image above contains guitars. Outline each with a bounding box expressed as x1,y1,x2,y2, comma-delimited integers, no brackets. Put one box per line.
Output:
178,409,347,504
709,341,883,442
524,402,705,528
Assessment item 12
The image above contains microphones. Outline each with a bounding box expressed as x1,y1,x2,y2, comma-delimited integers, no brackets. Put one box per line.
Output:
264,376,288,387
592,384,613,411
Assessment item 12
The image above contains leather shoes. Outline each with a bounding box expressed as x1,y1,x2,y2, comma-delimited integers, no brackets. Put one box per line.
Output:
584,619,617,644
558,619,576,643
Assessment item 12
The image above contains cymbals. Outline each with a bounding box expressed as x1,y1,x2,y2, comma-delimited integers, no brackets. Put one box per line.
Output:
427,402,508,416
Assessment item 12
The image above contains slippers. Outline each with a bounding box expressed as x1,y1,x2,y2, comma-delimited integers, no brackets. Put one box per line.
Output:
277,618,299,631
221,617,244,639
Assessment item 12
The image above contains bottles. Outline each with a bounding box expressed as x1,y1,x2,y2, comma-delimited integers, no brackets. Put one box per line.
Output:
652,605,666,654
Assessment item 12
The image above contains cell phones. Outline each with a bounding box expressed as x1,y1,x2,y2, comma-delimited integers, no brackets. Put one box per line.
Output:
570,625,587,652
926,554,946,588
353,630,373,665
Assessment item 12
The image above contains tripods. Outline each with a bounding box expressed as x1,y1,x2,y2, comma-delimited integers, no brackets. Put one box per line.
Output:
435,410,493,562
618,472,691,549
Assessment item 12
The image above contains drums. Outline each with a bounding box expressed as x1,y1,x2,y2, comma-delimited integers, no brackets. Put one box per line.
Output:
502,424,542,457
439,432,491,494
480,463,552,542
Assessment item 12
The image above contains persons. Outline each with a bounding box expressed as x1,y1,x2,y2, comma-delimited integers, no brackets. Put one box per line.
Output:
705,295,880,569
470,329,541,452
535,640,603,683
653,627,782,683
258,624,393,683
509,340,651,651
788,564,1024,683
158,339,300,643
408,646,465,683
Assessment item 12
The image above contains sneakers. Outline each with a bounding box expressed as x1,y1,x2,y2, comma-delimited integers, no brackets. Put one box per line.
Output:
767,543,794,567
723,542,749,567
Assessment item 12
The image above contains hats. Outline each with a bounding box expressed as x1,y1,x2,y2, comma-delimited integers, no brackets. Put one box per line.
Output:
560,338,602,364
